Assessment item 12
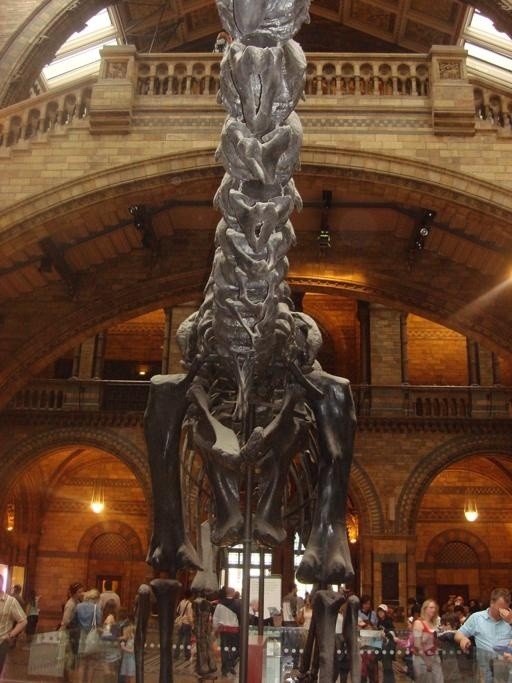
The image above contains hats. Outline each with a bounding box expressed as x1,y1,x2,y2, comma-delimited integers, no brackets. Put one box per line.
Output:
378,604,388,611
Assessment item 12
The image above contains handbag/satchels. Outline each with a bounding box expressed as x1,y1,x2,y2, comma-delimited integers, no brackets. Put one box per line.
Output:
84,629,100,655
173,615,184,631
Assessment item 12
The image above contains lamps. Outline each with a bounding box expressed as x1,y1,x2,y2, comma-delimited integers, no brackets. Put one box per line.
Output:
464,485,478,522
91,480,104,513
345,513,359,544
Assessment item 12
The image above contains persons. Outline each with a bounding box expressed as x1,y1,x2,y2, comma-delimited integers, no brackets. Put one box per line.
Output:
55,582,136,683
171,585,270,679
7,585,26,650
25,589,42,635
0,574,29,676
280,582,512,683
